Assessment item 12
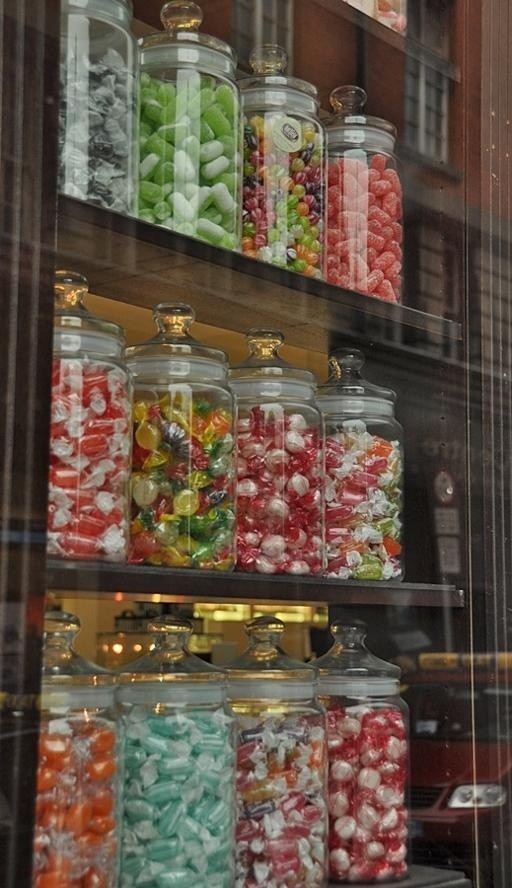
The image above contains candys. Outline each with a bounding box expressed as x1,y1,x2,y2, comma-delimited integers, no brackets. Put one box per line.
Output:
37,701,409,888
345,0,408,37
59,44,406,308
46,359,404,580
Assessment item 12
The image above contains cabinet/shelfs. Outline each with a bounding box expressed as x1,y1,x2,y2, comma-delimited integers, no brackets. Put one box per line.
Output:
1,1,512,885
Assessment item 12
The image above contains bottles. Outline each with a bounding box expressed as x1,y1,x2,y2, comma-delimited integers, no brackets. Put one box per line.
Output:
225,615,331,886
45,269,135,570
233,43,329,280
316,345,403,579
326,83,407,305
226,325,330,576
115,615,241,888
141,0,245,252
60,0,142,218
125,302,239,575
308,618,410,886
34,608,121,887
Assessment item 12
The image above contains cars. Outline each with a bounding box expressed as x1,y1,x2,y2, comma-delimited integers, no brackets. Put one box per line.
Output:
385,646,512,861
